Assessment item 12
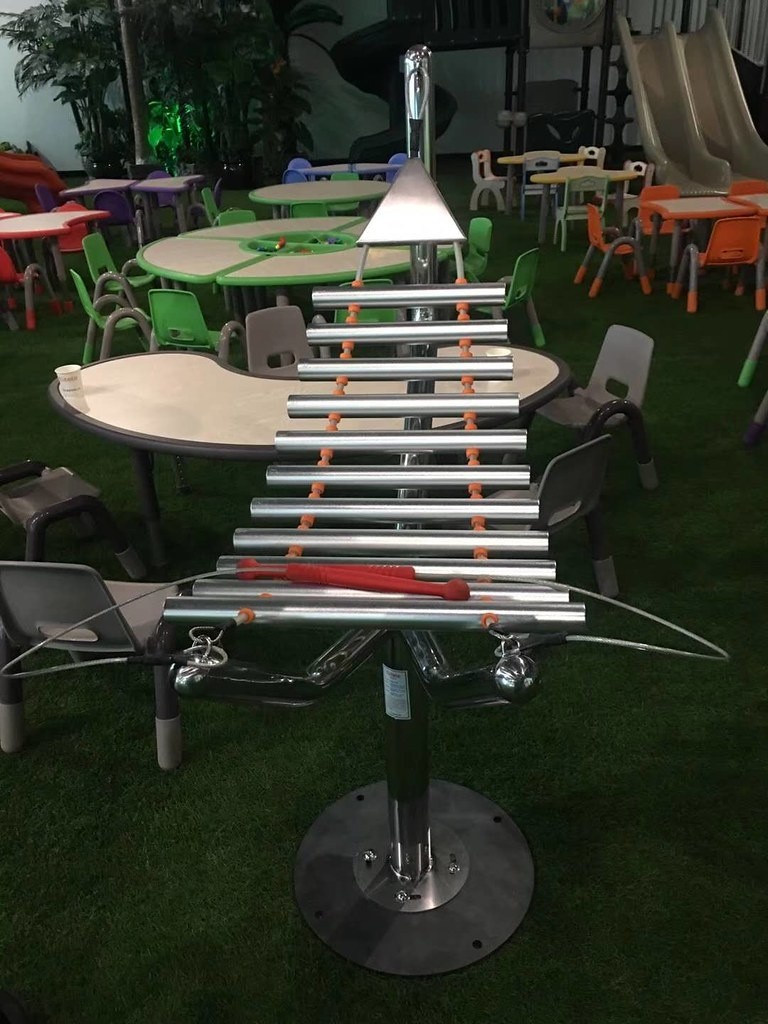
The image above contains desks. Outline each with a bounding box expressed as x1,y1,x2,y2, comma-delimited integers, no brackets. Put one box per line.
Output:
648,197,758,294
59,174,208,234
135,216,463,367
42,341,571,567
496,153,588,214
727,193,768,274
530,165,639,245
248,179,391,220
298,161,404,180
0,210,113,311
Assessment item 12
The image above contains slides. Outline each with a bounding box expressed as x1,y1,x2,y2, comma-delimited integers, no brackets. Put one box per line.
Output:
332,19,459,163
618,6,768,195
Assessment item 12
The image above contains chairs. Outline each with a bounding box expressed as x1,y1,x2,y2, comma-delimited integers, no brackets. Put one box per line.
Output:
0,153,408,327
469,149,520,212
149,288,246,364
621,184,693,280
577,146,607,205
595,160,655,228
0,560,245,771
574,203,652,297
729,180,768,275
553,174,609,253
500,325,659,490
0,459,149,580
68,268,152,365
245,305,314,379
473,247,545,346
440,215,494,320
671,216,767,313
461,431,619,598
520,151,561,220
312,278,411,358
